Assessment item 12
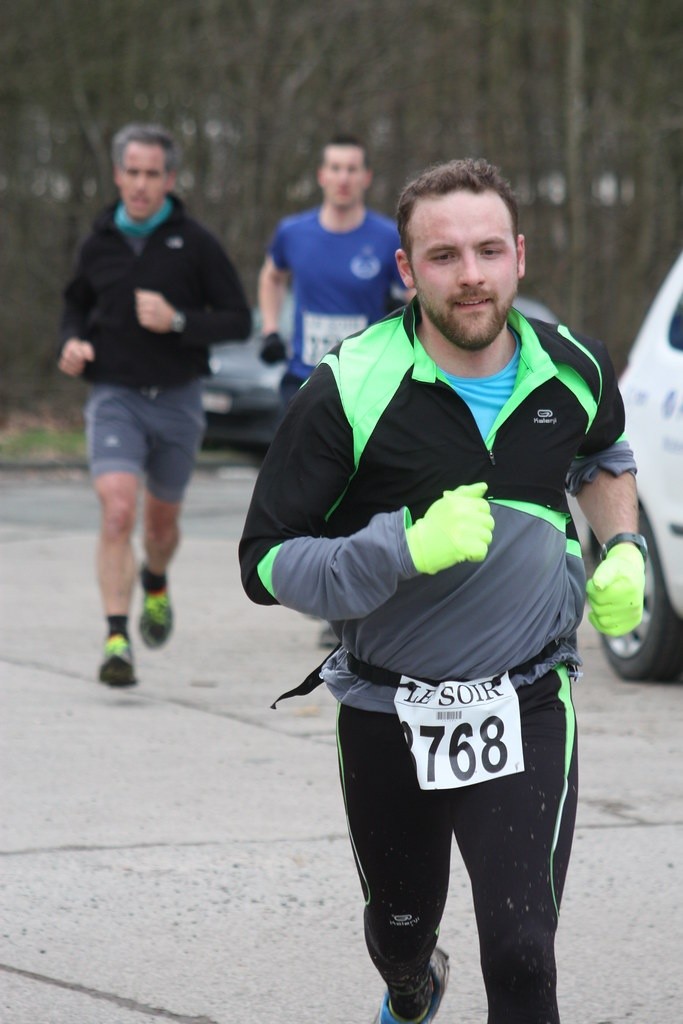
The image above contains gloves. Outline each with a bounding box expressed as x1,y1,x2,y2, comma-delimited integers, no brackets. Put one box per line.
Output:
585,544,646,638
406,482,495,575
264,333,285,362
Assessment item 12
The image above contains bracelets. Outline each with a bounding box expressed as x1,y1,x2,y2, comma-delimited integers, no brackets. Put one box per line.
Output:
173,312,185,332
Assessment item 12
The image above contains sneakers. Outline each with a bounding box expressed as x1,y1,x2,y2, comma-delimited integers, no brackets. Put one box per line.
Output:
139,563,173,647
373,947,449,1024
100,634,134,686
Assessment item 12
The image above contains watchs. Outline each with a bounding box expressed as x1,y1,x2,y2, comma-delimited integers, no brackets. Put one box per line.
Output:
600,533,649,563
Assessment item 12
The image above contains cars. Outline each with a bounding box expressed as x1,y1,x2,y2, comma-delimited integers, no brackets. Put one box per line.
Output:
580,250,683,687
198,305,291,456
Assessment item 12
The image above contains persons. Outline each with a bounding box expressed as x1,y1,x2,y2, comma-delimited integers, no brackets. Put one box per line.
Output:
238,159,646,1024
258,135,417,647
55,129,254,689
